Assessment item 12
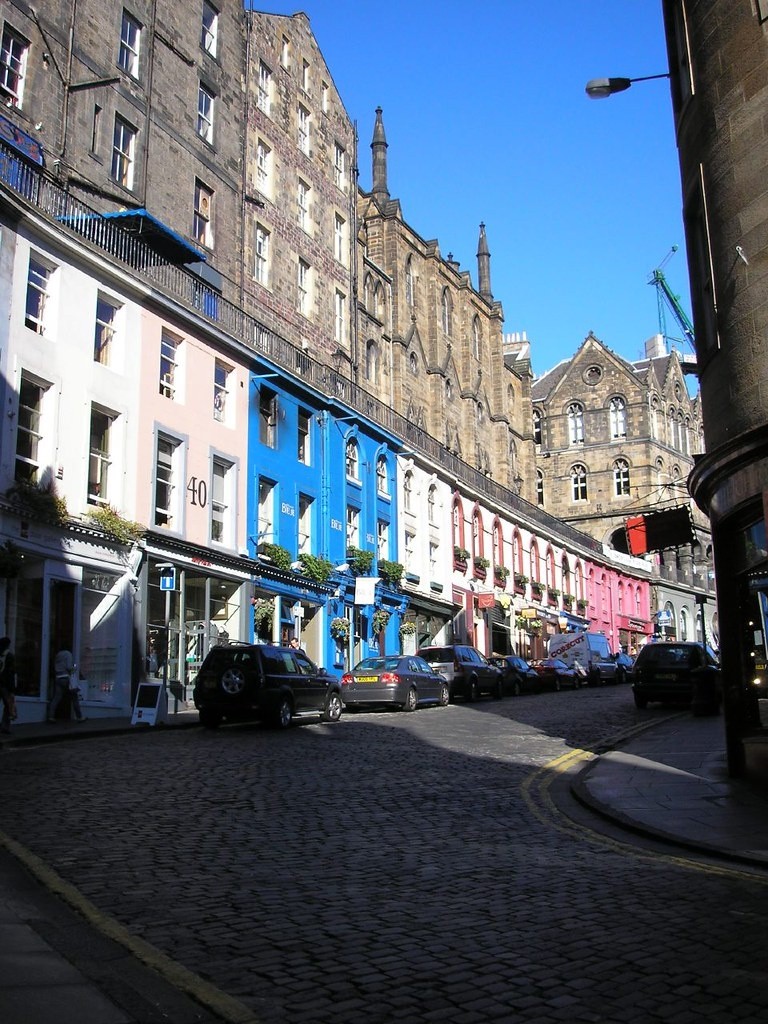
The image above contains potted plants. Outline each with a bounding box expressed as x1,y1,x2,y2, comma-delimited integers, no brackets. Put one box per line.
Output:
256,543,293,574
345,545,374,574
577,600,589,609
563,592,575,606
494,564,510,582
532,580,546,595
549,588,561,600
454,546,470,561
378,559,405,589
514,572,530,589
474,556,490,570
330,617,352,642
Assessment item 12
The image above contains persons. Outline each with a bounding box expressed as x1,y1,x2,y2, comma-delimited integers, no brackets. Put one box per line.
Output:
289,638,305,654
48,636,88,723
491,652,502,666
0,636,18,734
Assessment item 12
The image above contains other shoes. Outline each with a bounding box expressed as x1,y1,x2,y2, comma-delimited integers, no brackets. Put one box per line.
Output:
78,716,88,723
1,723,12,734
48,718,56,722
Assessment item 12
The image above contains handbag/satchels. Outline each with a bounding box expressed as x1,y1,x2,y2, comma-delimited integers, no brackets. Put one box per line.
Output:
68,670,81,692
6,696,17,720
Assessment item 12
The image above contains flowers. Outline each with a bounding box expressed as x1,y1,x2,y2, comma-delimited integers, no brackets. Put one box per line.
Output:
372,608,389,635
399,616,417,635
250,597,277,632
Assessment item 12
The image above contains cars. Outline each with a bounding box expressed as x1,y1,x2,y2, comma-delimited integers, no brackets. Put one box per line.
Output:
612,653,635,684
526,659,581,693
486,654,542,699
341,654,450,712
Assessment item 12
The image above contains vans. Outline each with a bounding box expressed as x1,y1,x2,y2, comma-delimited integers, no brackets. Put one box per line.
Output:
549,633,621,686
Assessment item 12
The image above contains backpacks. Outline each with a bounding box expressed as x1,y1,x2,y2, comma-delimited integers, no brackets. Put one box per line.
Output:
0,649,10,673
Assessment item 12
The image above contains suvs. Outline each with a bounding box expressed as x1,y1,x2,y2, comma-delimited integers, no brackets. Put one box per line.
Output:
415,644,504,701
632,637,723,709
193,644,343,729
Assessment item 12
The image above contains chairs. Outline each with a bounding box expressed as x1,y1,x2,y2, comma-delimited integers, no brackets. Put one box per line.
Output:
664,652,676,664
